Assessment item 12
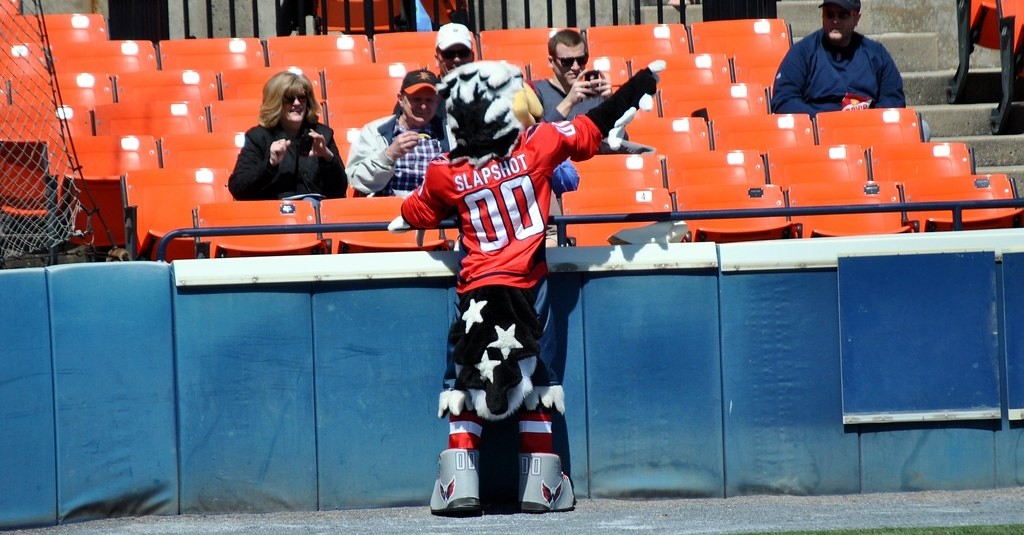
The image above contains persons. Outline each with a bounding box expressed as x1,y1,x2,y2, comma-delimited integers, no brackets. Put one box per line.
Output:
770,0,907,120
344,69,440,197
532,28,657,154
227,66,348,207
433,21,475,153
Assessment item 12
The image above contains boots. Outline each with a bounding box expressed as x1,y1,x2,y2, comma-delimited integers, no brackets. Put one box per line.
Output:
515,451,577,511
430,449,489,516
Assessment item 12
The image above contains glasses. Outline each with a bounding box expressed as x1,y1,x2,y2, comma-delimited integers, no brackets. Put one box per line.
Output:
283,94,309,103
553,53,589,66
823,9,857,19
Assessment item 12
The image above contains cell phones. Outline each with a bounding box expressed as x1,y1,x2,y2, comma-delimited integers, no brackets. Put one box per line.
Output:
585,71,599,97
298,135,314,157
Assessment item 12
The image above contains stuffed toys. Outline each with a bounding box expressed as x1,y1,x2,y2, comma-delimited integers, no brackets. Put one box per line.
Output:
386,58,667,514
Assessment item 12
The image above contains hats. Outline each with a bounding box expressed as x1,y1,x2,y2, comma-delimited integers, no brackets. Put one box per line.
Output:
400,69,439,95
819,1,862,9
434,22,475,52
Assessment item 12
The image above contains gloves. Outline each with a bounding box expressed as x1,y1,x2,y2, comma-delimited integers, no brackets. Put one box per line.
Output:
388,216,411,234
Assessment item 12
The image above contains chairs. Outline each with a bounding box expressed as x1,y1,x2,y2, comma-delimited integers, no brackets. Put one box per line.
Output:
0,0,1023,262
